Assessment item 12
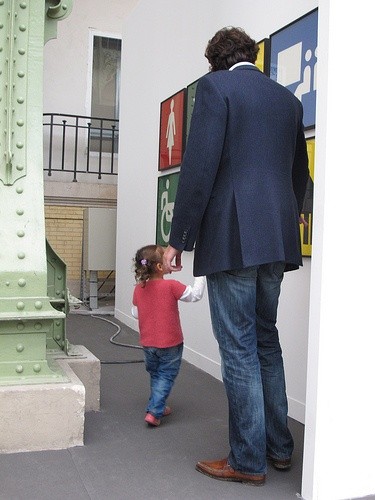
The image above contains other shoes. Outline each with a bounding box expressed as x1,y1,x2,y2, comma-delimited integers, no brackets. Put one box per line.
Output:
160,408,171,416
145,413,160,425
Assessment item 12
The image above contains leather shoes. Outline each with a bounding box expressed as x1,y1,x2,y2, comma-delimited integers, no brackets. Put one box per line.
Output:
195,458,265,487
268,459,291,469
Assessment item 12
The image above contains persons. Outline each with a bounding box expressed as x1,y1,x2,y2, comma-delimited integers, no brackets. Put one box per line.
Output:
162,25,308,485
132,244,206,426
